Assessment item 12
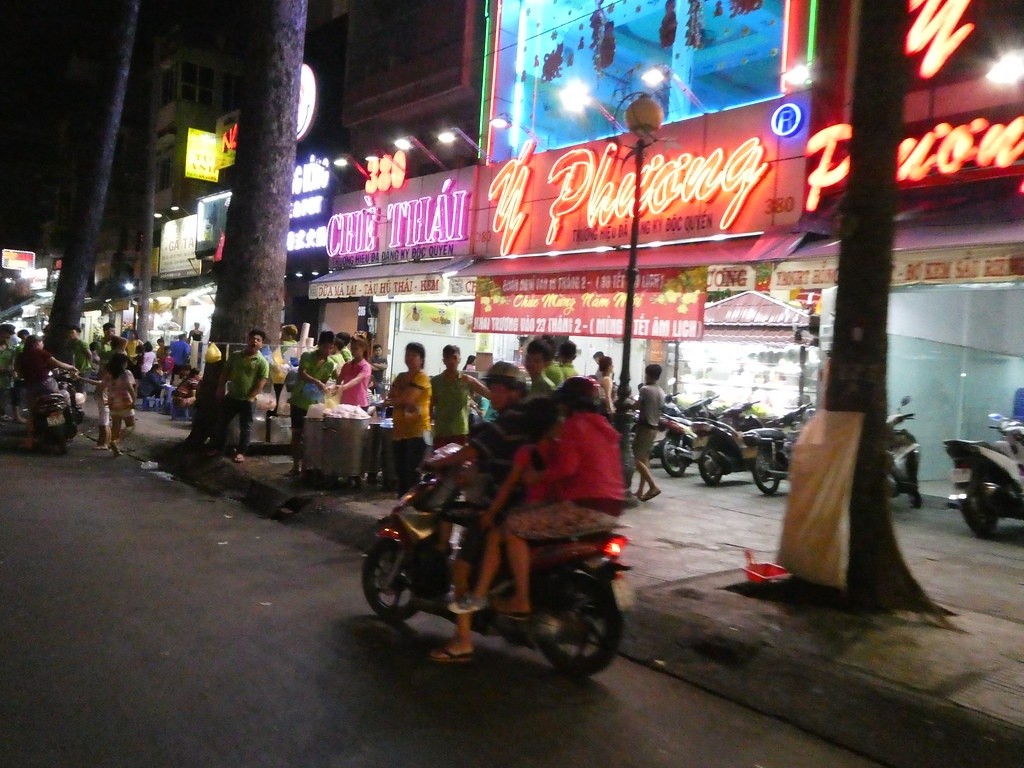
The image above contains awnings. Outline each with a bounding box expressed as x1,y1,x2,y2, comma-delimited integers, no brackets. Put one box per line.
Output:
310,196,1024,302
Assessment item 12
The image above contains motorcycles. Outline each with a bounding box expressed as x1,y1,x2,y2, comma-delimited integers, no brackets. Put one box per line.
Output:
886,394,923,507
613,378,816,495
944,413,1024,538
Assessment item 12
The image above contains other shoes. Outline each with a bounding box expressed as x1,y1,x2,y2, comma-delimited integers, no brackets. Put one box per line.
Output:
283,470,300,477
232,452,246,463
109,441,125,456
14,416,28,424
202,450,216,456
18,442,33,450
0,415,14,422
448,594,490,615
93,444,109,450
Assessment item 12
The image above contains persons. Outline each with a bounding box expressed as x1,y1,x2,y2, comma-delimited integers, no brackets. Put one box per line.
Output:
421,360,625,661
281,321,665,504
0,323,202,456
205,329,270,460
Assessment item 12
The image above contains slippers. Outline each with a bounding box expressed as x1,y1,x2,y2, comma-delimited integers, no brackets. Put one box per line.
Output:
491,596,530,621
425,643,475,662
641,488,662,501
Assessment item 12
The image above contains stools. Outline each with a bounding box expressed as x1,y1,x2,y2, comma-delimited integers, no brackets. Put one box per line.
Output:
137,384,191,420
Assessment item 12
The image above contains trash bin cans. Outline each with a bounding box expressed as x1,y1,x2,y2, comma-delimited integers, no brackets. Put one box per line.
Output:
301,416,325,471
368,424,394,490
322,417,373,491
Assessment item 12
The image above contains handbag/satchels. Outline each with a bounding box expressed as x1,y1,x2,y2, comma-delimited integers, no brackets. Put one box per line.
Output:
205,341,221,363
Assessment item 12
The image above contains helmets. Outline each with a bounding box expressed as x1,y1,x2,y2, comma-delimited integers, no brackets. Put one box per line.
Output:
478,361,526,389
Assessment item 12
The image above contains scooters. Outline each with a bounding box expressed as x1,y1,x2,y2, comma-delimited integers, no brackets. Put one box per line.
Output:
362,443,633,677
30,368,82,449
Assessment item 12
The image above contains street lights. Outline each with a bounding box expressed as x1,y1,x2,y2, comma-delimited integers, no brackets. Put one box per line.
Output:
614,92,663,467
